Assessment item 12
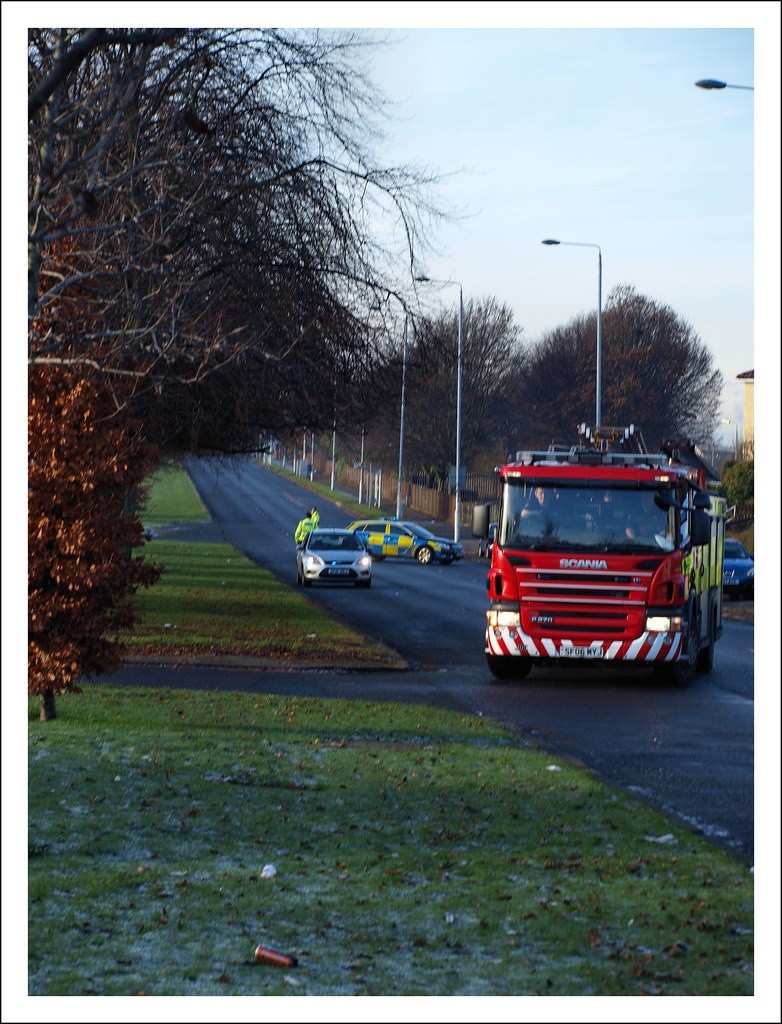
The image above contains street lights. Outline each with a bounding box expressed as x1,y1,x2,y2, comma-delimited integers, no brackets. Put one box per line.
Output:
542,238,602,428
416,275,463,545
370,305,409,521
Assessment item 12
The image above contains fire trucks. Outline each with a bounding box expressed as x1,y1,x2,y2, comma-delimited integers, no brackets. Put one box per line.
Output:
471,422,736,685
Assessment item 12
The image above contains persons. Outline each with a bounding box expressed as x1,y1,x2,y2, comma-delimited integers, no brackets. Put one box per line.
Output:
294,506,320,544
520,487,565,522
624,492,668,548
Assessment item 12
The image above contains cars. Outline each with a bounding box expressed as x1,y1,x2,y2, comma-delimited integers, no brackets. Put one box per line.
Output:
345,519,464,566
722,537,754,602
478,522,498,559
296,528,374,588
